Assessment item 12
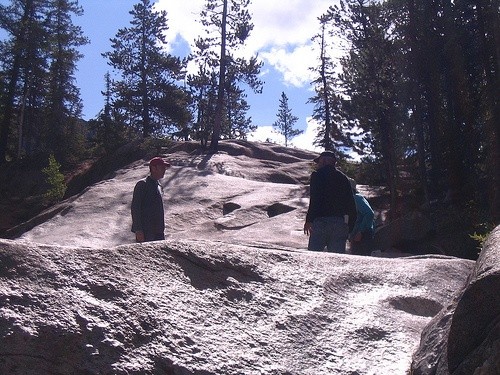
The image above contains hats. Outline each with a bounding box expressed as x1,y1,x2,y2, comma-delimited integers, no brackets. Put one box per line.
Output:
149,157,170,172
349,178,356,190
314,150,336,165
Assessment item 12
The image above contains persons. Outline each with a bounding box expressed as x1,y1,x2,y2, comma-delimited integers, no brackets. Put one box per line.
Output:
304,152,356,254
348,178,375,256
131,157,171,243
